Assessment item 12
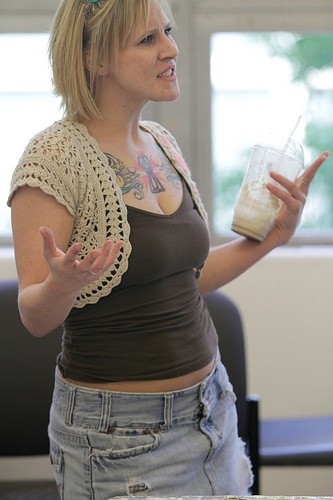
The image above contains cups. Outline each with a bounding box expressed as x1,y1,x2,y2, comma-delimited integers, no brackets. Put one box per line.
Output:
233,137,304,243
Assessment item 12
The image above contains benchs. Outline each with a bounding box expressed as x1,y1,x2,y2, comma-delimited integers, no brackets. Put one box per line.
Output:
0,280,260,495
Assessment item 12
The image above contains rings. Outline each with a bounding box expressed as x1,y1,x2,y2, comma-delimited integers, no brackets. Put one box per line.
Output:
301,178,311,185
90,269,103,275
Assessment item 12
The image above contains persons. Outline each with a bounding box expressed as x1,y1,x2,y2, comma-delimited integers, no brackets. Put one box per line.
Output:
6,0,330,500
